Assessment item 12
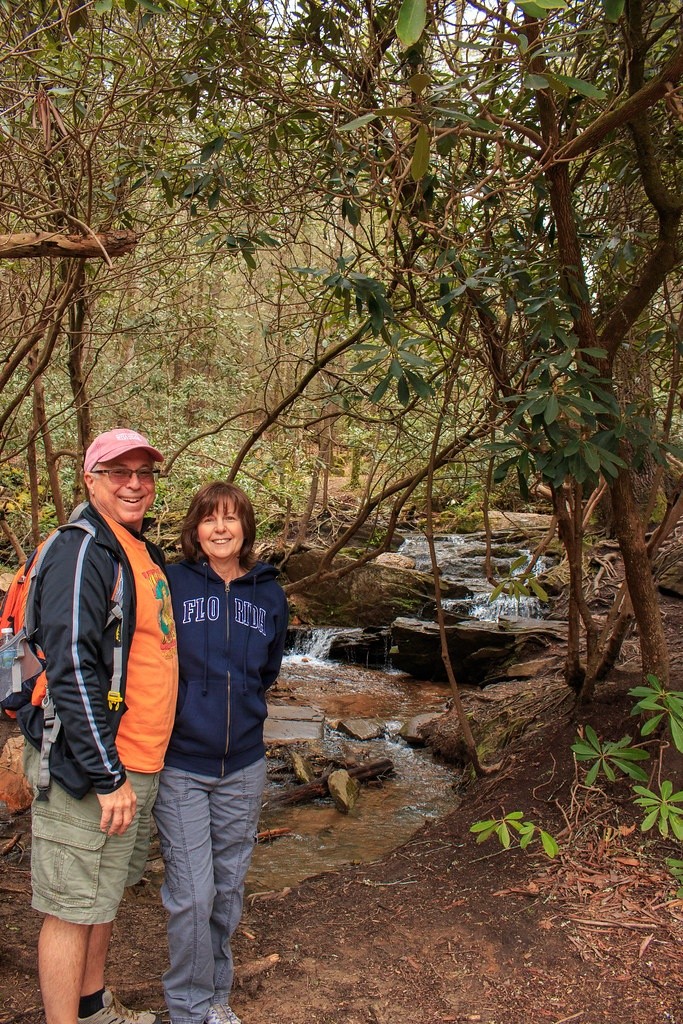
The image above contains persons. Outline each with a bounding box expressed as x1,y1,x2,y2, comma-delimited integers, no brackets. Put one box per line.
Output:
18,430,181,1024
147,479,287,1024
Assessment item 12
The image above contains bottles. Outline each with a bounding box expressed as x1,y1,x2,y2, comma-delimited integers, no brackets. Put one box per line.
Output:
0,628,22,701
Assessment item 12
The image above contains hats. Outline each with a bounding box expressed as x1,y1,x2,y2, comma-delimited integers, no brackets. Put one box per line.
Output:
83,428,165,474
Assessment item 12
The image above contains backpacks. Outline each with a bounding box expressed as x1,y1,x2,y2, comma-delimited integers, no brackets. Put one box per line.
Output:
0,518,124,792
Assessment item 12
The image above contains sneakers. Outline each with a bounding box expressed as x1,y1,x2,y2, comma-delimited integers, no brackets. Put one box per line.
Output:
77,988,161,1024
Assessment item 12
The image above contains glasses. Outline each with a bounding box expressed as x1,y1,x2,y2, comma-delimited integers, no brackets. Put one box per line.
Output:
92,464,160,482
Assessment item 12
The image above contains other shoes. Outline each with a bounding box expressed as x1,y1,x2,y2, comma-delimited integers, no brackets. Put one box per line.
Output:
204,1005,240,1024
